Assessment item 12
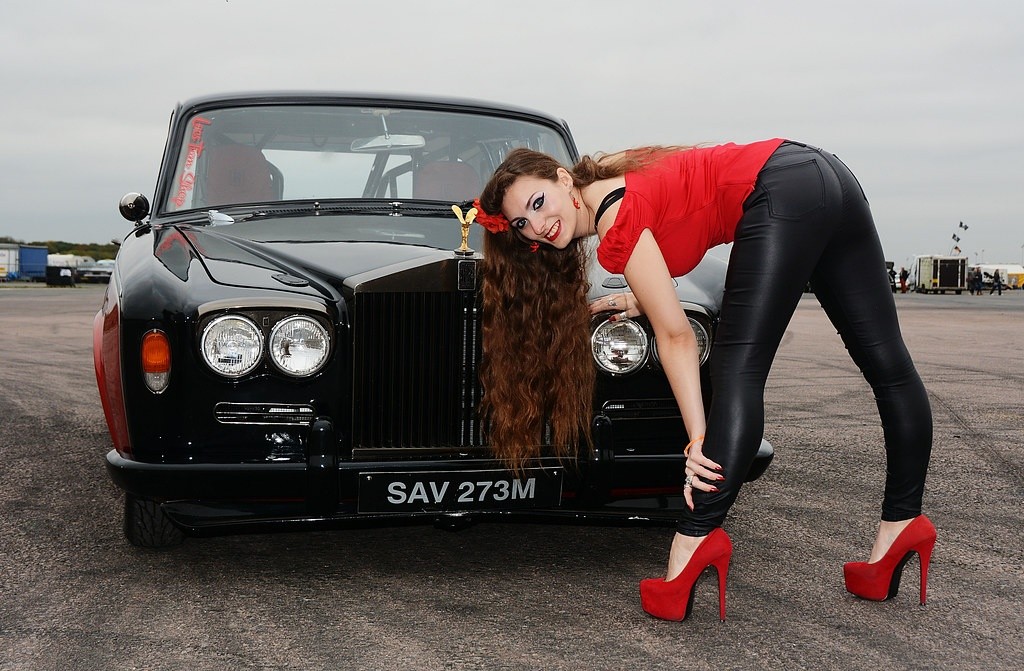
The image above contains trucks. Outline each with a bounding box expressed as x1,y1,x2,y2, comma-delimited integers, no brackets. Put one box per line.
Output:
48,253,95,268
905,255,968,295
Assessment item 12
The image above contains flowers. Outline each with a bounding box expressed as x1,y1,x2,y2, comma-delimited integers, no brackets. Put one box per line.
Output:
472,198,510,233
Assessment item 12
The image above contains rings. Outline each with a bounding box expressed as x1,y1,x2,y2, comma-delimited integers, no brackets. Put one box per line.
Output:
608,298,617,306
620,312,628,320
685,475,696,485
684,485,692,489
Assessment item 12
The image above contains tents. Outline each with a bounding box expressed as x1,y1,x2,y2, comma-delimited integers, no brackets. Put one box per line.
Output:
968,265,1024,284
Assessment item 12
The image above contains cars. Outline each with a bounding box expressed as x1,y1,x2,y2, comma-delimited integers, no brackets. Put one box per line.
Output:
93,91,780,547
97,259,116,267
895,275,903,291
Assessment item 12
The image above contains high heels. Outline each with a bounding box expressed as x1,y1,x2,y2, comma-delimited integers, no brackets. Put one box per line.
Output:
844,513,937,606
640,527,733,622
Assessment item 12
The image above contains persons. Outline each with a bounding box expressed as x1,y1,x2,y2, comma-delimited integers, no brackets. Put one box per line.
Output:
969,268,983,295
989,269,1003,295
888,268,897,293
899,267,908,294
476,139,937,621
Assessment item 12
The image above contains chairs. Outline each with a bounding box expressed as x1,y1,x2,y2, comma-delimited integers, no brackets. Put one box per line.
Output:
413,162,479,208
207,143,277,206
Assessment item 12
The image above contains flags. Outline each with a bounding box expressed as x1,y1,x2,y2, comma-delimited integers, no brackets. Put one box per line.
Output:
953,246,961,255
952,233,960,243
959,221,969,230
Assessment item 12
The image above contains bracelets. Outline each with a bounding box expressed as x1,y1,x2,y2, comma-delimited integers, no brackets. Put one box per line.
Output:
684,436,705,457
